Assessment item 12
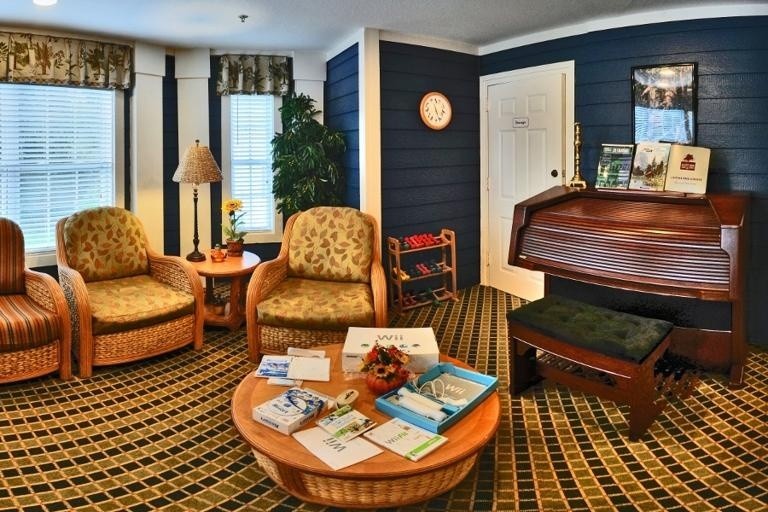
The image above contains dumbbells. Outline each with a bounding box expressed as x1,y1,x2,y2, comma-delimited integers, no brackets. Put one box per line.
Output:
394,260,442,280
399,234,440,250
403,288,441,306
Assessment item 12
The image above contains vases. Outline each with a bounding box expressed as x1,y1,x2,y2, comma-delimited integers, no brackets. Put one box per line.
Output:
363,369,406,392
226,238,244,257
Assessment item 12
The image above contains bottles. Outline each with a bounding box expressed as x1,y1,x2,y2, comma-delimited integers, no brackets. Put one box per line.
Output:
209,244,228,263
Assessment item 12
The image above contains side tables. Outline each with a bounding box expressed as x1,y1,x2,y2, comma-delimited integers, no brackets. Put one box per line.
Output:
176,250,262,330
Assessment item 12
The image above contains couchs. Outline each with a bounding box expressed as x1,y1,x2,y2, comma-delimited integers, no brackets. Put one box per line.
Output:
246,204,387,363
54,206,206,379
0,217,73,385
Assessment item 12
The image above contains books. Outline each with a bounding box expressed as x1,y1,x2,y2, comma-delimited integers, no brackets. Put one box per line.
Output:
363,417,448,462
314,405,377,443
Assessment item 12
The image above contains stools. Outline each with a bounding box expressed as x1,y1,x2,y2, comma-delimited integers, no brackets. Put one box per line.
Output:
505,296,676,441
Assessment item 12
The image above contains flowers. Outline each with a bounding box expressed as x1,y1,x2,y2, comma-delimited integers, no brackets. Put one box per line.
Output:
219,197,249,241
357,344,417,378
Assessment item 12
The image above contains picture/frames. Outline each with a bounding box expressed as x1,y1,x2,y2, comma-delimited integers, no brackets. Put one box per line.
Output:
629,62,698,147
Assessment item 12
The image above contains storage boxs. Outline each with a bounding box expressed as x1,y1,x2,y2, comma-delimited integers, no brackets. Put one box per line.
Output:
339,325,441,374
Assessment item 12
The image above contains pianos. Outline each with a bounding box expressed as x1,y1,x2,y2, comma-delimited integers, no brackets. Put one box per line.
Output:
508,185,748,391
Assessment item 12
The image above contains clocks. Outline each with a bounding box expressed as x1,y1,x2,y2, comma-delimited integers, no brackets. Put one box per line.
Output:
419,92,453,130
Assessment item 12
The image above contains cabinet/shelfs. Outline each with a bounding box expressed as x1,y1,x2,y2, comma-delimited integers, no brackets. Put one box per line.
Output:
387,229,460,314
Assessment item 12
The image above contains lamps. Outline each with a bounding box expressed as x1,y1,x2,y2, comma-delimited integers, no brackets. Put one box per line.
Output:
169,138,224,263
